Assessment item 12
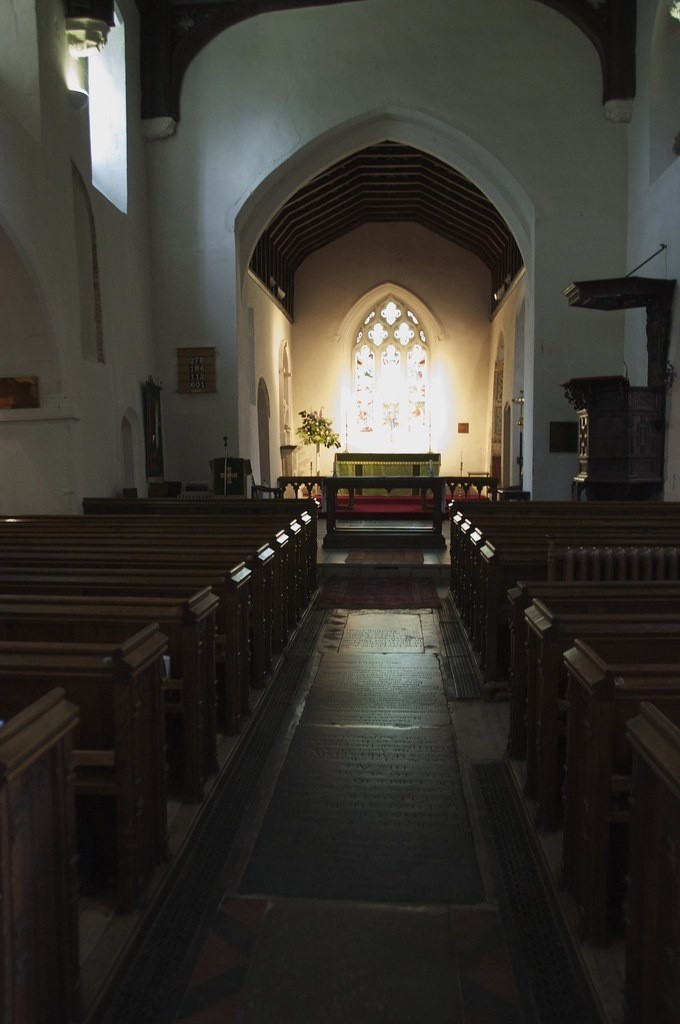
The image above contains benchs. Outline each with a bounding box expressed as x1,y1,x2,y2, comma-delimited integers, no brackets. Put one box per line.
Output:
523,597,680,834
469,526,680,653
0,510,312,609
460,516,680,633
0,528,290,655
625,700,680,1024
0,688,86,1024
82,497,320,594
0,519,303,628
452,510,680,610
0,542,276,692
507,580,680,758
561,637,680,952
0,620,174,915
0,585,222,805
0,561,252,740
480,538,680,679
447,498,680,591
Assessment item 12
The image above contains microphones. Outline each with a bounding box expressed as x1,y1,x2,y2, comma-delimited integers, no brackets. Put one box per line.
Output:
223,436,228,447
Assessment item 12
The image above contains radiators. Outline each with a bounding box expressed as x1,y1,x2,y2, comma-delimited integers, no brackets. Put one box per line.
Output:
546,545,680,580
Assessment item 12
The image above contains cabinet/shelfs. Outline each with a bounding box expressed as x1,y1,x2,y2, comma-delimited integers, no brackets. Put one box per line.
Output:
561,375,664,502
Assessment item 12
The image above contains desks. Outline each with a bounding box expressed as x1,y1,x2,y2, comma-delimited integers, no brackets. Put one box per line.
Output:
319,476,449,552
333,453,441,497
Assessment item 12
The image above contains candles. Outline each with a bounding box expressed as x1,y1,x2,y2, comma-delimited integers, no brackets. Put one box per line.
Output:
460,448,463,462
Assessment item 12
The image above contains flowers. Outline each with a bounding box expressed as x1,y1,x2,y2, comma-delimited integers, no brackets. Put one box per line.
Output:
295,410,341,449
